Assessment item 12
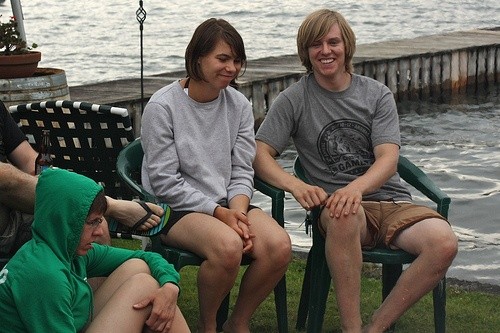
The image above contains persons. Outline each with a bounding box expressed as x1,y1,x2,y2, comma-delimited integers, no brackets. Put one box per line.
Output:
249,9,457,333
0,99,175,270
0,166,191,333
141,18,292,332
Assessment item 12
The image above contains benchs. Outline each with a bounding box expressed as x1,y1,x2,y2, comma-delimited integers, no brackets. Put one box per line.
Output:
9,100,136,201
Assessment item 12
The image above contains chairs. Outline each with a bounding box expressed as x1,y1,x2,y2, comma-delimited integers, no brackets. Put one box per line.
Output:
292,153,451,333
115,136,288,333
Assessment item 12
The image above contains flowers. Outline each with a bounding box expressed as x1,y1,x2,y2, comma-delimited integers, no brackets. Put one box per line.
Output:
0,14,38,56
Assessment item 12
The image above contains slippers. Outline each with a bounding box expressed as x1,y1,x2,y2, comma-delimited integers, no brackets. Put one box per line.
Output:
106,198,174,237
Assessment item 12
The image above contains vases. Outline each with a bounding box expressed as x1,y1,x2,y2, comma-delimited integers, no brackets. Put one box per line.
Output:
0,51,42,78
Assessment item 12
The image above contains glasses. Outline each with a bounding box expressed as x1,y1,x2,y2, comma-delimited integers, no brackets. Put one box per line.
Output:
85,217,103,228
